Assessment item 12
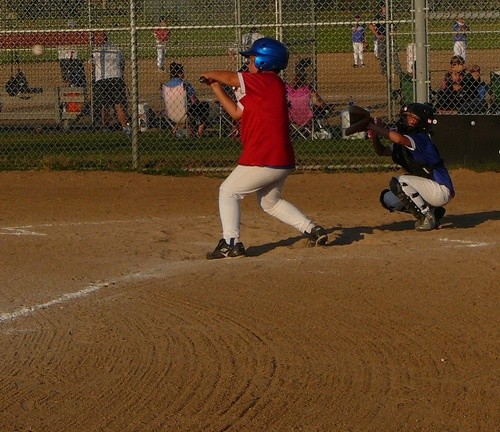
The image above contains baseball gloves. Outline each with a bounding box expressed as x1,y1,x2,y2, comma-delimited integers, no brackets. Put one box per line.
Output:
345,106,375,136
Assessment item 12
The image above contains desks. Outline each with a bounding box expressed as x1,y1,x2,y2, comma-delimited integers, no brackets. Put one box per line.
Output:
321,98,352,129
197,97,228,133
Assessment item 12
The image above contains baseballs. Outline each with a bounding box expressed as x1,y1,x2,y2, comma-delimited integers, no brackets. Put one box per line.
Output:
32,44,44,56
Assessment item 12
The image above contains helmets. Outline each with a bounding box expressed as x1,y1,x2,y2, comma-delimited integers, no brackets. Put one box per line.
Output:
238,37,289,71
397,102,435,134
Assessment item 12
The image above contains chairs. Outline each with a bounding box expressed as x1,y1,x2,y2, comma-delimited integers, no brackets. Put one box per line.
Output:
160,96,199,139
220,103,240,137
291,99,314,143
56,87,90,128
489,71,500,113
400,71,413,105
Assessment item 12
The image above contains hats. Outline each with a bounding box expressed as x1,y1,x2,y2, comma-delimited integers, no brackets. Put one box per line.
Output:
91,33,108,47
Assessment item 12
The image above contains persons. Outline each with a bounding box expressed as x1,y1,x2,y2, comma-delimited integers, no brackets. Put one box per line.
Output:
153,16,170,71
161,63,209,137
290,69,328,136
367,103,456,231
200,37,328,260
88,32,127,132
368,0,402,75
436,56,487,113
351,15,365,68
58,20,86,87
452,16,469,61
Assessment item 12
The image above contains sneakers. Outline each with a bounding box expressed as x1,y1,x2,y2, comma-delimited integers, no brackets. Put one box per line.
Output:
415,206,446,227
414,209,437,232
121,122,132,135
307,224,328,249
205,238,247,259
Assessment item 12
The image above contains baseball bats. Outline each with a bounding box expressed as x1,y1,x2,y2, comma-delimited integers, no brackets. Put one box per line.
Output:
200,76,210,84
